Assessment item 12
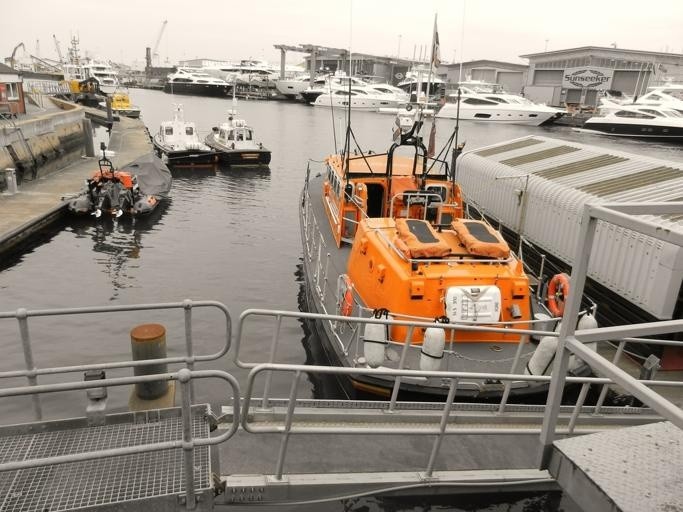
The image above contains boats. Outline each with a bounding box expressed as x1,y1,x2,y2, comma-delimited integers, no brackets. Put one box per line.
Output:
310,71,410,110
162,67,232,98
58,77,139,117
155,103,214,168
67,141,172,216
435,79,568,126
580,88,683,142
205,83,271,167
299,79,599,402
62,65,118,93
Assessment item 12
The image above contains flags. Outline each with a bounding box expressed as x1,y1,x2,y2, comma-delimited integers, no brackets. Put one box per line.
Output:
431,24,443,68
427,122,435,158
